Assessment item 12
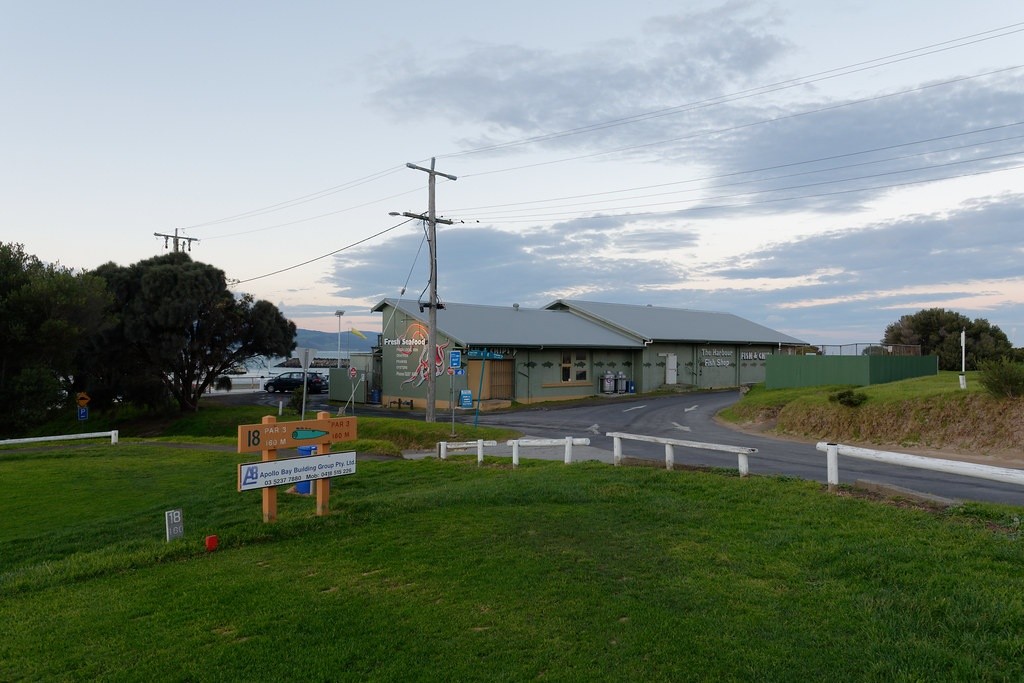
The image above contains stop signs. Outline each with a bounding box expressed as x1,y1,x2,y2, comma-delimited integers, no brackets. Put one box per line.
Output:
350,367,357,377
446,367,454,375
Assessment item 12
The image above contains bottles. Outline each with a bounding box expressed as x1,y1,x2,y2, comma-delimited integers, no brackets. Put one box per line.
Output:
615,371,626,393
603,371,614,393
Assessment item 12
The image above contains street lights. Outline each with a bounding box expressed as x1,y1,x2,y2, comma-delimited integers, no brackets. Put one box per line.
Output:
334,309,346,369
388,209,440,422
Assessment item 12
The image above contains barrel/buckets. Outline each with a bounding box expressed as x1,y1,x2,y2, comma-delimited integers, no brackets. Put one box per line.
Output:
627,381,635,393
296,446,311,494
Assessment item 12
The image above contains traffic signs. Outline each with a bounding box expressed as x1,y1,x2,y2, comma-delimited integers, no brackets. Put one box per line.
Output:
467,350,484,357
487,352,504,360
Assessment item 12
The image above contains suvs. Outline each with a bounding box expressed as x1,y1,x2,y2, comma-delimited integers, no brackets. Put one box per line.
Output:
263,371,329,394
